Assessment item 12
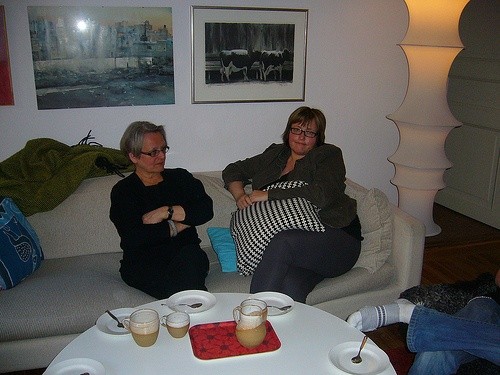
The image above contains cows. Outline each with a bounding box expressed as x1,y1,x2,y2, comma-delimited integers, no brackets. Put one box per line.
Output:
256,48,294,82
217,48,262,83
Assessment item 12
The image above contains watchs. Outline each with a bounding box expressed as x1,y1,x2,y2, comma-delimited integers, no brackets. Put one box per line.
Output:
166,206,174,220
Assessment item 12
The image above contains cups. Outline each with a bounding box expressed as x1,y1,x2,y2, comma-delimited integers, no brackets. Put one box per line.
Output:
122,308,160,347
160,312,190,338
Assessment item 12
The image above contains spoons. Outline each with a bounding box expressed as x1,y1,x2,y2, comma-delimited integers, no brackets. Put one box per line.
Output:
161,303,202,308
105,310,125,328
351,336,368,363
267,305,292,311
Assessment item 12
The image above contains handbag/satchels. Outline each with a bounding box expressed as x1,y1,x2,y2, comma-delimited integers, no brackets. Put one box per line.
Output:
0,197,44,290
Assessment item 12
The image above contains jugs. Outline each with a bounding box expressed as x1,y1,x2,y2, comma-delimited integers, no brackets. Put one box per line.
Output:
233,299,268,348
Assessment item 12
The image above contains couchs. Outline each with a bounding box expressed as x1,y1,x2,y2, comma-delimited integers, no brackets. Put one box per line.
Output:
0,168,426,372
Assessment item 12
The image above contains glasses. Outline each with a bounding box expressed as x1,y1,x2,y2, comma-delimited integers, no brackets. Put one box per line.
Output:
289,127,317,138
136,145,169,158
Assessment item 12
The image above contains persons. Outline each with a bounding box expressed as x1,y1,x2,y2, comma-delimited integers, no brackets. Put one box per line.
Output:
346,270,500,375
107,120,215,299
220,106,364,304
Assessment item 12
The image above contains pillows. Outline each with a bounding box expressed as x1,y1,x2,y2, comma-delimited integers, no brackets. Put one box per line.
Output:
0,198,44,291
209,197,327,277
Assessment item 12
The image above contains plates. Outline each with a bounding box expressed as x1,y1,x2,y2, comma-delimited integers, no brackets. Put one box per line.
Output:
245,292,294,316
96,308,136,336
167,290,217,313
328,342,390,375
44,357,106,375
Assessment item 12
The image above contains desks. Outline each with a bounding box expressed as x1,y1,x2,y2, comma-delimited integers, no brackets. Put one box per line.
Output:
41,292,397,375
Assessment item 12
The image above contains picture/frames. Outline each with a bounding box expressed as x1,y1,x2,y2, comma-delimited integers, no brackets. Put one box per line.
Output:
190,5,309,104
